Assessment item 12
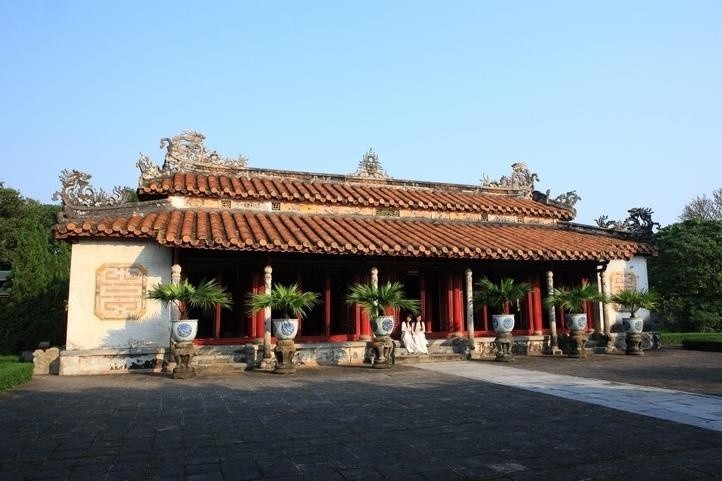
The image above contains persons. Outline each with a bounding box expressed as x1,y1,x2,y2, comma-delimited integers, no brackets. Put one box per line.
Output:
400,313,421,354
411,314,429,354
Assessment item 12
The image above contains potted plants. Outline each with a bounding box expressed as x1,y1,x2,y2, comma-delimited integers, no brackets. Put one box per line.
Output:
469,275,534,334
609,287,660,334
244,280,324,340
144,276,241,342
543,282,608,332
344,279,422,335
